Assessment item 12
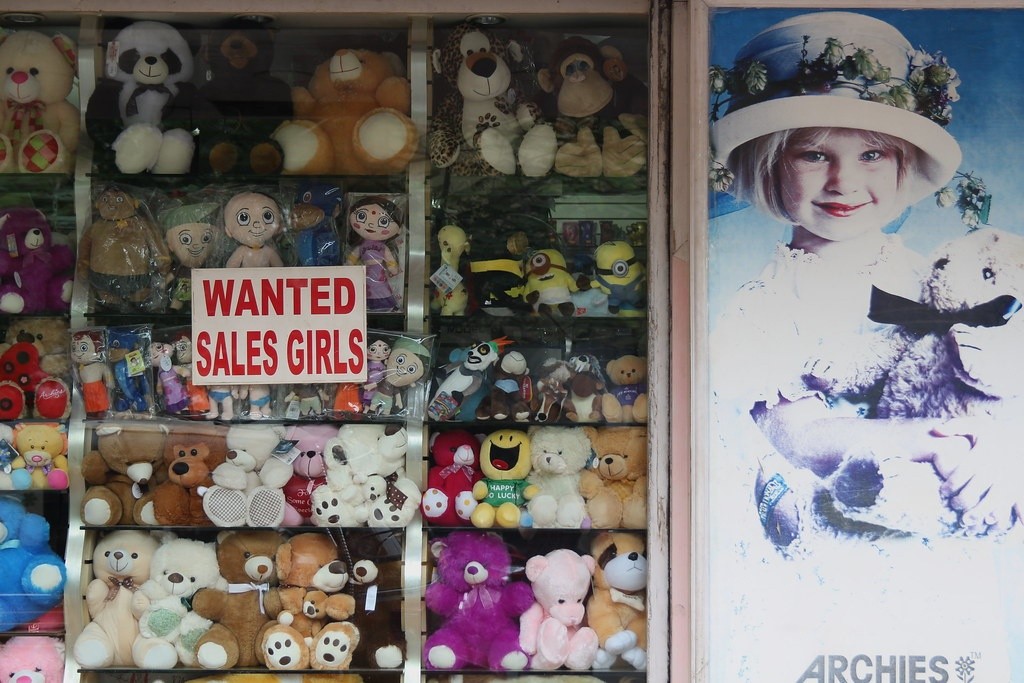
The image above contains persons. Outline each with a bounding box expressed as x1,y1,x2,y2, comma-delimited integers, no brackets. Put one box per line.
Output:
70,180,428,419
709,10,1023,529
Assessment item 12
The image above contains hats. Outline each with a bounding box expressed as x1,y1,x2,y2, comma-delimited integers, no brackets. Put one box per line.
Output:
710,12,963,206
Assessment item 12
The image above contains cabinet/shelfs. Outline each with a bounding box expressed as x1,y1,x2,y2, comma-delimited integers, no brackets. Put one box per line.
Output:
0,0,670,683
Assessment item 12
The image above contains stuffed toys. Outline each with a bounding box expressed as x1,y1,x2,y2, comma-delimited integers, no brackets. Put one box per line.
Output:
753,226,1024,559
73,425,420,683
0,202,73,683
424,15,647,683
0,24,419,176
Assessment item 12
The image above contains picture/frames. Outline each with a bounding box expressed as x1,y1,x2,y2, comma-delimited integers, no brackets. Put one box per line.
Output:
689,1,1024,682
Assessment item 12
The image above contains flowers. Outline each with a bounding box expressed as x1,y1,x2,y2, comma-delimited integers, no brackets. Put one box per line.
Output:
707,36,987,232
